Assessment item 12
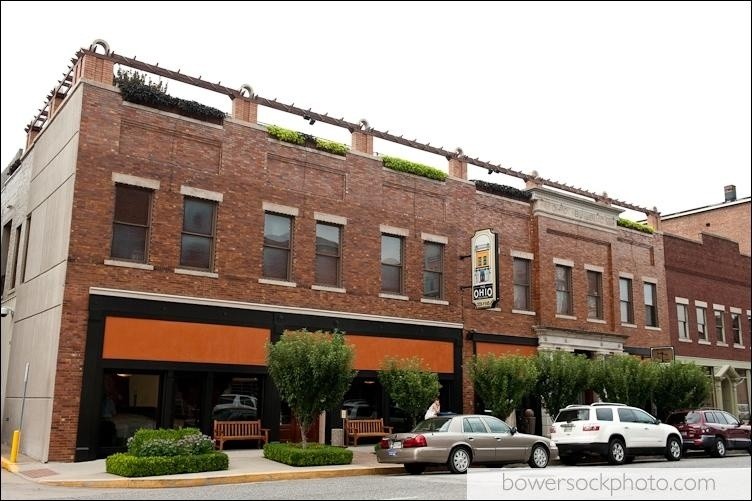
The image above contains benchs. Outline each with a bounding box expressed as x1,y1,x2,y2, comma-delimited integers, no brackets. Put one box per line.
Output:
345,417,394,446
214,419,270,452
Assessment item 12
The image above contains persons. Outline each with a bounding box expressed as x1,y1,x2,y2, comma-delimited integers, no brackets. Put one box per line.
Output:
173,391,189,428
101,393,118,421
424,397,441,420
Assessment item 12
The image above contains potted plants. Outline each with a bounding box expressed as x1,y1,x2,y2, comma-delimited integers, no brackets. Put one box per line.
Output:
329,409,345,446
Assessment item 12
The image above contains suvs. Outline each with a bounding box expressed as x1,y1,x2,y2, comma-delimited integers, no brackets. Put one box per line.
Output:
549,403,685,466
665,408,751,458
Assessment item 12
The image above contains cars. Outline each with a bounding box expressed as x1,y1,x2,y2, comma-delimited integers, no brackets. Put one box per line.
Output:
342,402,378,421
212,395,258,414
377,414,560,474
